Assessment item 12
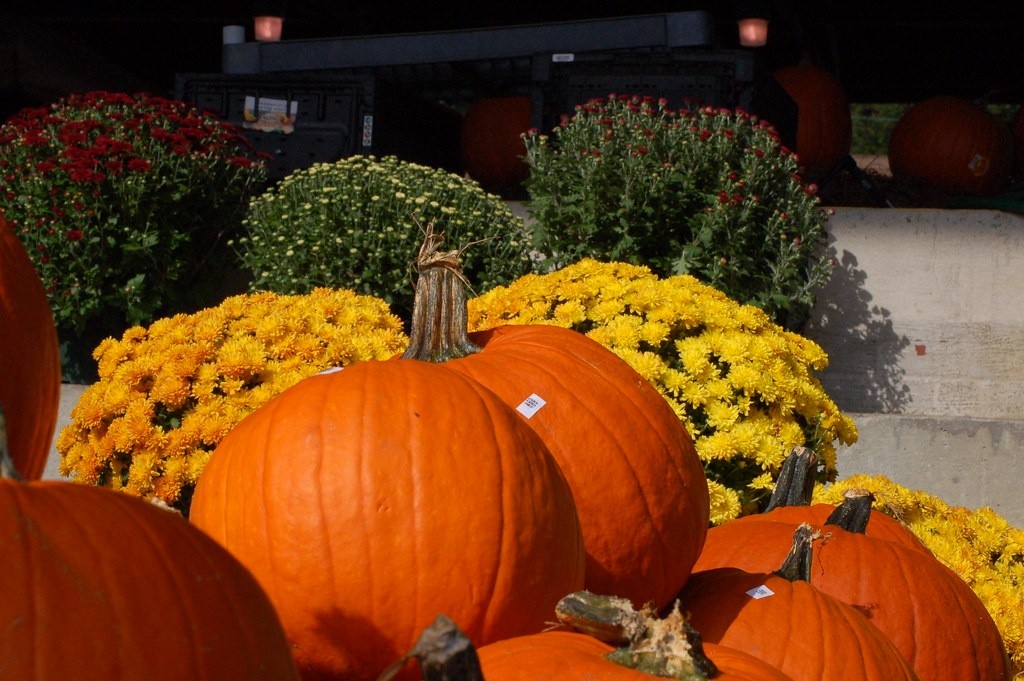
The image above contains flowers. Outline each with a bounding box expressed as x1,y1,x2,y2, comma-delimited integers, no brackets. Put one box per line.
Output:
521,90,836,334
224,154,535,331
807,473,1023,681
0,89,273,385
465,256,861,526
57,286,413,516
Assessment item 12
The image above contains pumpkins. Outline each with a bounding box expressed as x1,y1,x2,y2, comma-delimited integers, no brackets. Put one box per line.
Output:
770,58,851,171
0,203,1012,681
885,98,1024,198
459,89,536,188
